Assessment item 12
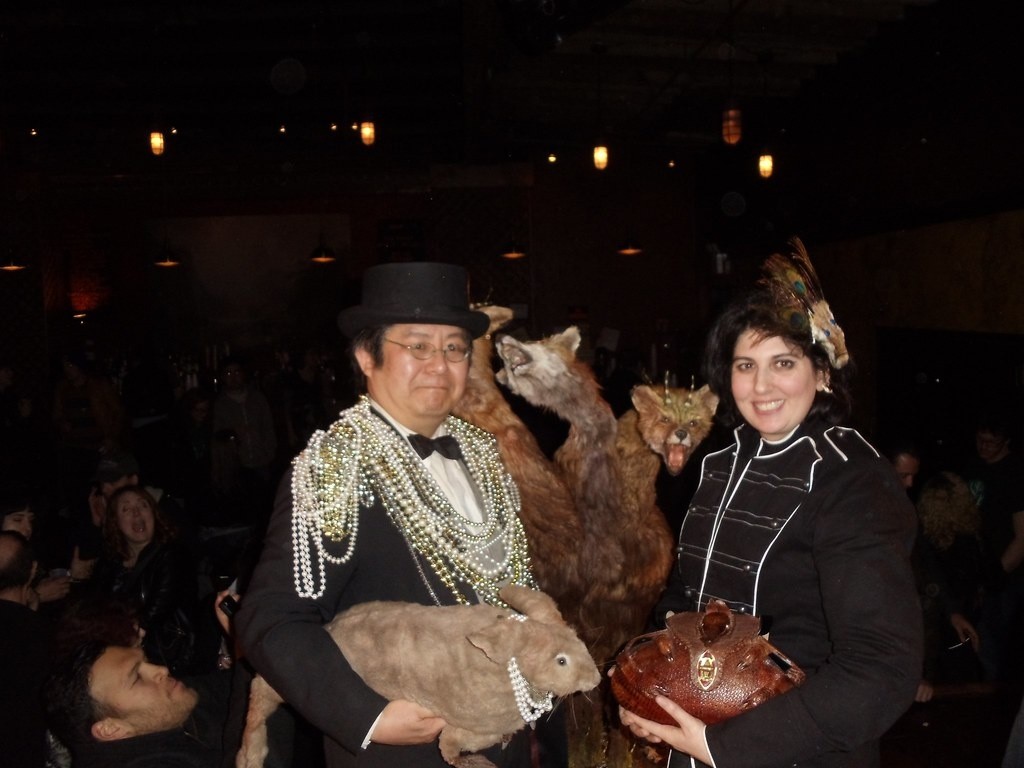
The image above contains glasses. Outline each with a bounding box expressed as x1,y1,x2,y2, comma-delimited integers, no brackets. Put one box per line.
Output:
382,339,476,364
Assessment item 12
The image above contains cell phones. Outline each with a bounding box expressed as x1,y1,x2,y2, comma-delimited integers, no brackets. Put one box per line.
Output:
219,595,243,623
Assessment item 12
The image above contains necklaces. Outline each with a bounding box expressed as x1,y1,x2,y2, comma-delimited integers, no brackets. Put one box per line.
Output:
288,402,556,725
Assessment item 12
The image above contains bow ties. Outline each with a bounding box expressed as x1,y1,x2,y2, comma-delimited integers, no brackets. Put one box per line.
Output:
409,432,463,461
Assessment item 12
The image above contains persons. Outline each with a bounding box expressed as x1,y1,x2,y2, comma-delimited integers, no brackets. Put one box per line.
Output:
876,416,1024,768
0,352,278,768
607,296,925,768
227,258,571,768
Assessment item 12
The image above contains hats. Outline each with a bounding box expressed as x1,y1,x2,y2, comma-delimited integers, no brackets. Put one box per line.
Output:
338,263,490,339
